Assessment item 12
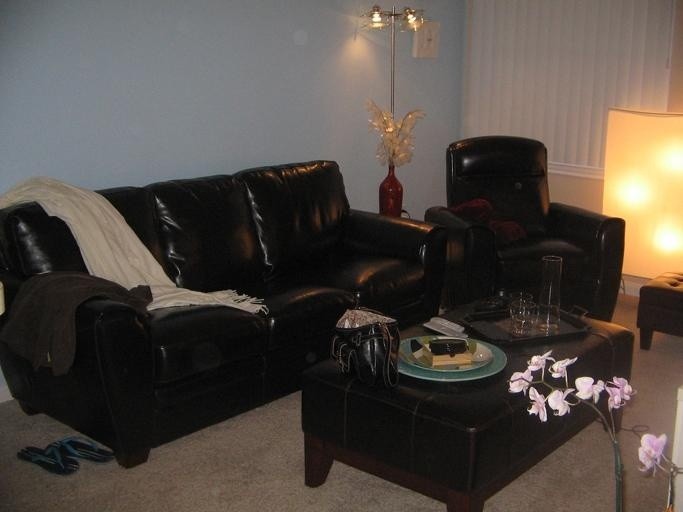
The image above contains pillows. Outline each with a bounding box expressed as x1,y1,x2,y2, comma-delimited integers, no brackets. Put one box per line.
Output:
449,196,528,250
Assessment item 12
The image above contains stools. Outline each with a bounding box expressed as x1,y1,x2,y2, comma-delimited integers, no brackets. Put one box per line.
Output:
635,272,683,351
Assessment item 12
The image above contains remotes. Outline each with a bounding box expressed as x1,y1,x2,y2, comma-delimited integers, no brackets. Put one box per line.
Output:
430,316,464,333
423,321,468,339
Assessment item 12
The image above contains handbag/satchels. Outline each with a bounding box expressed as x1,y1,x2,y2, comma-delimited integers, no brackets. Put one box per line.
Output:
328,307,401,388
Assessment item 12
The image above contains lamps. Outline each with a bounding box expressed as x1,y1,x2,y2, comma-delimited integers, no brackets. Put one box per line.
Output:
357,4,427,222
599,106,683,282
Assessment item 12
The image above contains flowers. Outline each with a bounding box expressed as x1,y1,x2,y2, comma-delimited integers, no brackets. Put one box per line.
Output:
506,349,683,512
361,95,432,169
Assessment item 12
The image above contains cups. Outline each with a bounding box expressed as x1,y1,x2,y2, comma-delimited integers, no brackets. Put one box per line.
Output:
508,298,537,338
537,254,562,334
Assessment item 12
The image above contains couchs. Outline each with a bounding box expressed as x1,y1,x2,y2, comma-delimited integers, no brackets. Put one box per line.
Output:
0,159,450,470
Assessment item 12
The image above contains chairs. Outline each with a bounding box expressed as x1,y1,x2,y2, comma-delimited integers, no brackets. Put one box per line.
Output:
423,134,626,324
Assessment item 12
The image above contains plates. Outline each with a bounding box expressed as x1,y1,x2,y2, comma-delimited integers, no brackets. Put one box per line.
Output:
397,334,493,372
396,334,507,382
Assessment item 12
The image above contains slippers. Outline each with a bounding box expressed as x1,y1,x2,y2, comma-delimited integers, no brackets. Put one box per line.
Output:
17,436,113,475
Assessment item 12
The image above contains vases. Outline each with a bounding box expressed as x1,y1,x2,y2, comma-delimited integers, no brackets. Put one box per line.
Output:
377,163,405,219
